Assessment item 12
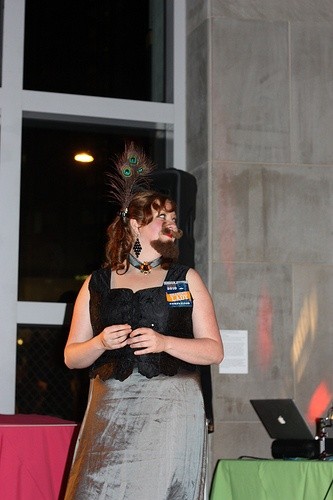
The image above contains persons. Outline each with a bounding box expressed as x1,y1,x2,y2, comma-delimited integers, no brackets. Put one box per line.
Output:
64,141,224,500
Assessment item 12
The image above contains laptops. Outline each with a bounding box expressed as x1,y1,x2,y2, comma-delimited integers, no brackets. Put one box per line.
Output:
250,399,320,440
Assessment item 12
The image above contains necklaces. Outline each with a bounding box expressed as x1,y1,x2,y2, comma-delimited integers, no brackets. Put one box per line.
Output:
128,254,162,276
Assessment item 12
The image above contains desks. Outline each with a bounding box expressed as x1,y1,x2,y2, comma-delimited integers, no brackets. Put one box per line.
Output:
209,458,333,500
